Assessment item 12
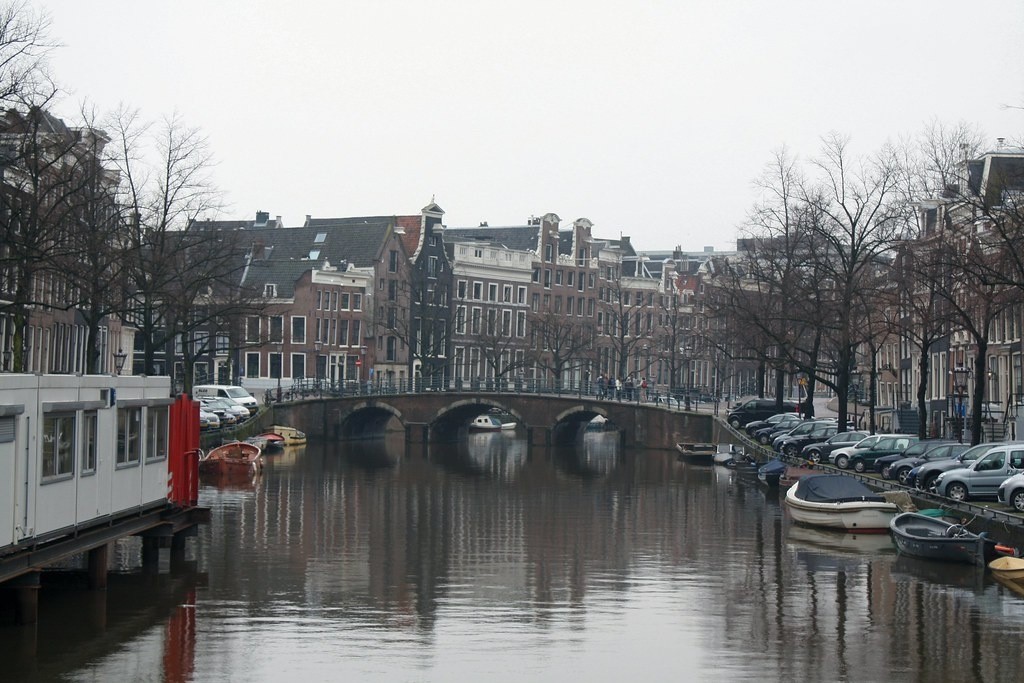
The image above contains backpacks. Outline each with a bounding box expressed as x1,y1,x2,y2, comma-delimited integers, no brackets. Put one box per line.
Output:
642,380,648,389
598,376,605,388
610,378,616,385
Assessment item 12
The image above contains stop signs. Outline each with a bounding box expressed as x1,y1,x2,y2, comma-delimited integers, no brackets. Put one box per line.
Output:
355,358,363,367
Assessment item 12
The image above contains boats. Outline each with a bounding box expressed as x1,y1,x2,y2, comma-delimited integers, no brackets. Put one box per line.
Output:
986,555,1024,580
757,460,824,490
784,473,898,533
263,423,308,444
199,443,261,475
713,442,748,464
471,415,502,429
251,433,286,447
238,435,269,449
888,511,998,560
502,422,517,430
675,440,717,459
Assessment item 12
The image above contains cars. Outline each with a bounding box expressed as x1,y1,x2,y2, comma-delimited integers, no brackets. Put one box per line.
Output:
781,426,861,457
801,431,871,463
745,413,854,457
828,433,917,470
191,397,221,429
933,444,1024,502
996,469,1024,513
852,436,919,474
872,439,974,488
199,397,251,429
916,441,1024,498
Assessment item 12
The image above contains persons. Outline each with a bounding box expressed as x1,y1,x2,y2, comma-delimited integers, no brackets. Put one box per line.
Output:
596,374,648,403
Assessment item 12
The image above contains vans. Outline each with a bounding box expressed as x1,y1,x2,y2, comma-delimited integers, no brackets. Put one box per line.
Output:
192,384,260,416
727,398,801,430
